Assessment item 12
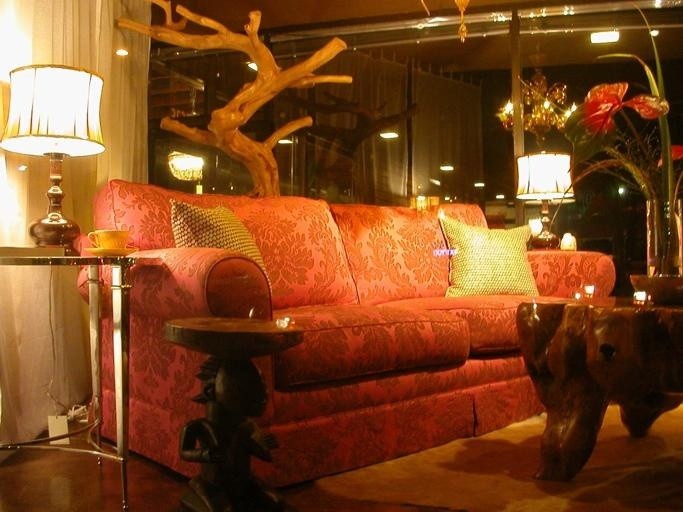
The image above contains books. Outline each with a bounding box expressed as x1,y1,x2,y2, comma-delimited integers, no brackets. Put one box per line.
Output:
0,246,65,258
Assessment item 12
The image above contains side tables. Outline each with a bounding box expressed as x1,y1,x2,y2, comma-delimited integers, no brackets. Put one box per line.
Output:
159,314,308,511
0,255,163,511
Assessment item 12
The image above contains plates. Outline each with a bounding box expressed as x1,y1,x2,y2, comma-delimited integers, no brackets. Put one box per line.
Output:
84,248,137,256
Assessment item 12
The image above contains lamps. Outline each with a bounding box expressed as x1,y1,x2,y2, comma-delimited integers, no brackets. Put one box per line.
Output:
515,149,575,249
408,194,440,212
166,150,207,184
491,17,582,150
0,63,108,255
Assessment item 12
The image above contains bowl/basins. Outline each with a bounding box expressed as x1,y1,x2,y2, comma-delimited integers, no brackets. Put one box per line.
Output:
629,273,682,305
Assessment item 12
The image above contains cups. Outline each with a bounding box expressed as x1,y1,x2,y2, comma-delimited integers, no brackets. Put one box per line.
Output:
87,230,131,248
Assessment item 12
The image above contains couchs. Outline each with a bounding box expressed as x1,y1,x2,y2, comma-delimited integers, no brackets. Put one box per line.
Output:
76,177,617,487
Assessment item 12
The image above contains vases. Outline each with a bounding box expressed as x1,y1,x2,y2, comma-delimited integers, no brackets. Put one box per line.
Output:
643,197,682,273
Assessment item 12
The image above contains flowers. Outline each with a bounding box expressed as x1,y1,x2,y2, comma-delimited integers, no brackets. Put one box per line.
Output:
547,0,683,275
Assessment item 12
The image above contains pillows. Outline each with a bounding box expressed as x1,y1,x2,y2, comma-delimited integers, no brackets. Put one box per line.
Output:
436,211,542,297
161,199,274,298
